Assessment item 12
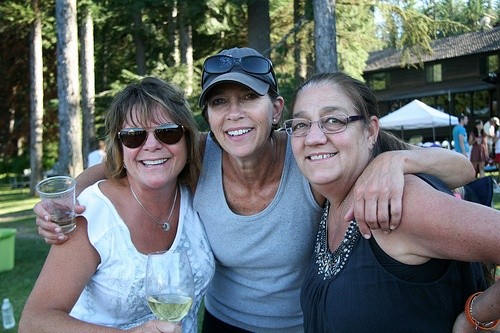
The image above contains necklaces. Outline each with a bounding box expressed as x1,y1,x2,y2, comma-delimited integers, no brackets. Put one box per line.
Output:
127,178,178,232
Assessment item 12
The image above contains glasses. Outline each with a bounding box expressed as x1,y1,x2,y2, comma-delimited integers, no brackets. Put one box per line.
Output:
283,114,374,138
200,55,276,89
118,124,184,148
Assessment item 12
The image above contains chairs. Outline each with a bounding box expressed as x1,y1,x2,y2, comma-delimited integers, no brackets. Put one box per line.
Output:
475,154,498,178
462,175,500,207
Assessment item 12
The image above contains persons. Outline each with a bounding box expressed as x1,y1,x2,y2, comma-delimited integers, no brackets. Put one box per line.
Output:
85,136,106,167
34,46,476,333
283,72,500,333
452,112,500,178
18,75,217,333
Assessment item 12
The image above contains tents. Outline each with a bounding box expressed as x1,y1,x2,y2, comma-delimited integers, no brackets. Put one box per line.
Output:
378,98,458,143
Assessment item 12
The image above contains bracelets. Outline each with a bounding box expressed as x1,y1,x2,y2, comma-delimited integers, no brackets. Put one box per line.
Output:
466,291,500,331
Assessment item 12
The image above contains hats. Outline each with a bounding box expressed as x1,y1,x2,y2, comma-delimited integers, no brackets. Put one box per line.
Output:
198,47,278,108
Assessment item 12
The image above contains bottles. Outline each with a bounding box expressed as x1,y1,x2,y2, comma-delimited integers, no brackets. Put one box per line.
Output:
1,298,15,329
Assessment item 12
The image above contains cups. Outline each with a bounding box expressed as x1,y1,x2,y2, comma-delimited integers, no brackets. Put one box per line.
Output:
35,175,77,234
146,250,196,323
477,140,481,145
